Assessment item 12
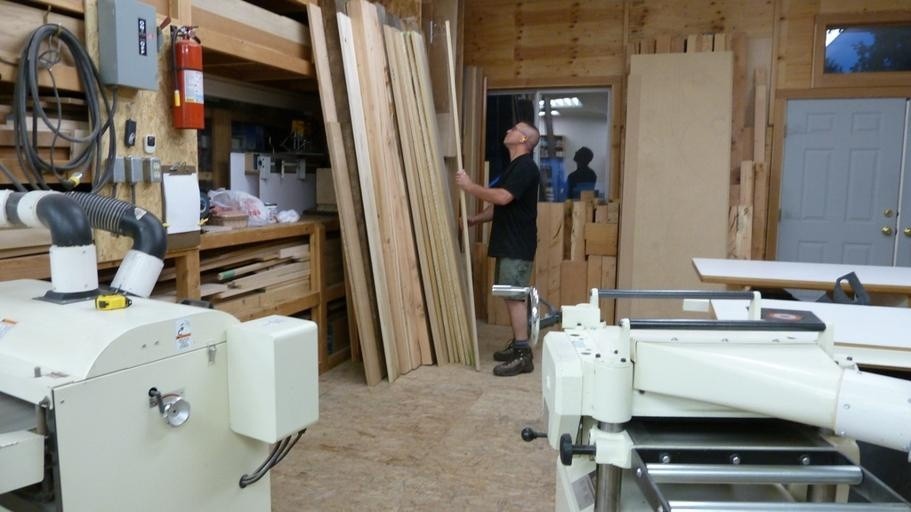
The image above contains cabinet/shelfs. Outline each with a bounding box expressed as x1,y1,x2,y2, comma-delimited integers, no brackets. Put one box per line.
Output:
98,212,350,377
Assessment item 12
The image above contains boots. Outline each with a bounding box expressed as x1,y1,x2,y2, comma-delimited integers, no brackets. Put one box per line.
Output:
494,348,534,376
493,338,533,361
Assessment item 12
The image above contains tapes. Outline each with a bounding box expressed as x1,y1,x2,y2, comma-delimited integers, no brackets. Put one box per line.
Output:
200,191,210,218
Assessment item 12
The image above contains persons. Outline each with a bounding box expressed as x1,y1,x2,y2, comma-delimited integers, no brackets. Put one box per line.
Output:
563,145,598,199
454,119,541,380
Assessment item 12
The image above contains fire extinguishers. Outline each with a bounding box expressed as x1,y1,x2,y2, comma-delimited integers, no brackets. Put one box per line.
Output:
171,26,206,129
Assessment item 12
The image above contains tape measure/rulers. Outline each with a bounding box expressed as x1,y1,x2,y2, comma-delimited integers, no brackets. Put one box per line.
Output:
94,292,133,310
